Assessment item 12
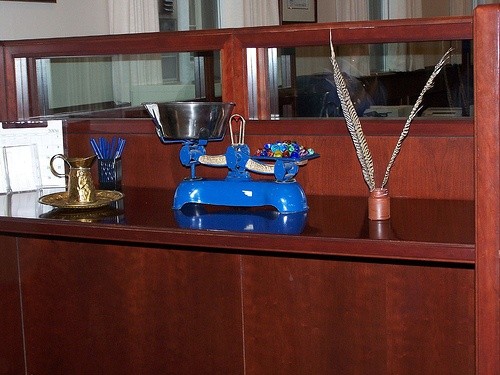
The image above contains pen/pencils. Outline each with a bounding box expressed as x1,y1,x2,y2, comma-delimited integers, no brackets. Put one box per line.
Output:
90,136,126,182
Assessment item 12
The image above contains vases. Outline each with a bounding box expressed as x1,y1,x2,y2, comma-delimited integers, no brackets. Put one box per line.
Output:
367,187,392,222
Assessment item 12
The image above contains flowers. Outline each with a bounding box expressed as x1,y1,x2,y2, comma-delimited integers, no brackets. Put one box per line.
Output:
329,25,455,193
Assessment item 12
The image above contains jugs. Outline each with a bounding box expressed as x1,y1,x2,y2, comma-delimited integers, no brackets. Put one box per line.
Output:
51,154,99,204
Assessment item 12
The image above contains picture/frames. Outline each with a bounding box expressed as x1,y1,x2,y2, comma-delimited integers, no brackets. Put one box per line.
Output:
278,0,317,27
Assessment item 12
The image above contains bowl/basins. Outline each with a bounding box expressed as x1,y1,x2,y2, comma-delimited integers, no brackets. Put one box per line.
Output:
141,98,236,144
